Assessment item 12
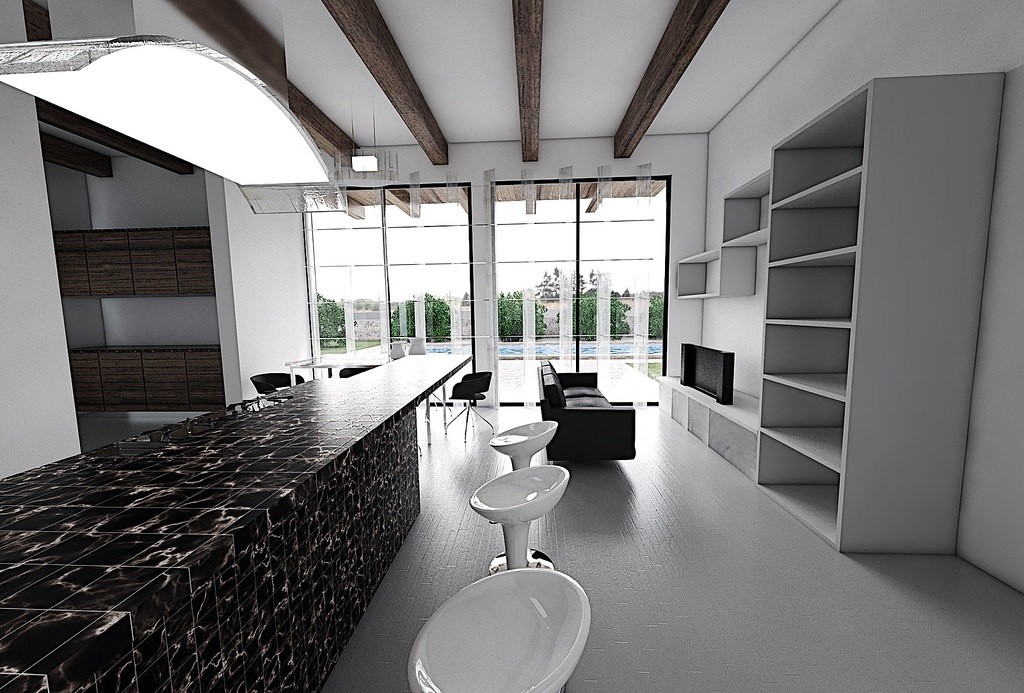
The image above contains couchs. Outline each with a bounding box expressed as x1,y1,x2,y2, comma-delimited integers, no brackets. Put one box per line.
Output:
536,360,636,462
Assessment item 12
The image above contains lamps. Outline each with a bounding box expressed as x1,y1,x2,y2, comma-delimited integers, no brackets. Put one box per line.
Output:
352,111,379,172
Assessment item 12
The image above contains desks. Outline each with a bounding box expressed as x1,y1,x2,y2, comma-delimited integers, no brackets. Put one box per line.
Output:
0,354,473,693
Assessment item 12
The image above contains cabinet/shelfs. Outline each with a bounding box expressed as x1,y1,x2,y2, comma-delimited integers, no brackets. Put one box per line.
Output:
656,72,1006,555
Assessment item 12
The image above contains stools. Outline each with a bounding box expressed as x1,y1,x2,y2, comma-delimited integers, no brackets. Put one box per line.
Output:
469,464,559,574
490,421,558,470
445,371,494,443
408,568,592,693
249,373,304,395
